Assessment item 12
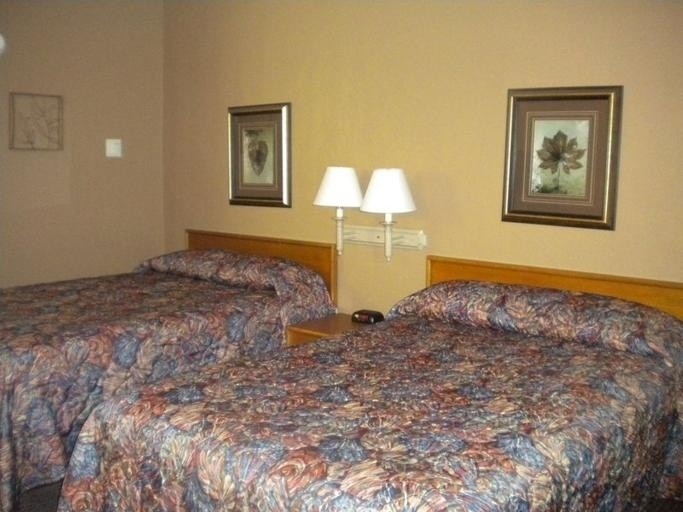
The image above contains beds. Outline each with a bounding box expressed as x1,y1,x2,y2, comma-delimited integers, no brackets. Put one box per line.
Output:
0,229,338,512
54,255,683,512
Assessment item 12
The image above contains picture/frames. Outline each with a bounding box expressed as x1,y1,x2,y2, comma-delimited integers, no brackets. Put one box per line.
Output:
227,103,293,208
501,85,623,231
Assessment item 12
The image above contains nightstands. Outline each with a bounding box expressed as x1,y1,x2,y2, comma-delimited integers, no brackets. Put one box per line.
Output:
285,312,373,349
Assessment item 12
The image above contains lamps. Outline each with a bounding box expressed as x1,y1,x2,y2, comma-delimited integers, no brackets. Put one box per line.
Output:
313,166,363,256
358,169,427,261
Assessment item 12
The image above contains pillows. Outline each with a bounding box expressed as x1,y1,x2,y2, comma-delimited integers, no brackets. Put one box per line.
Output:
386,278,683,371
136,247,334,340
